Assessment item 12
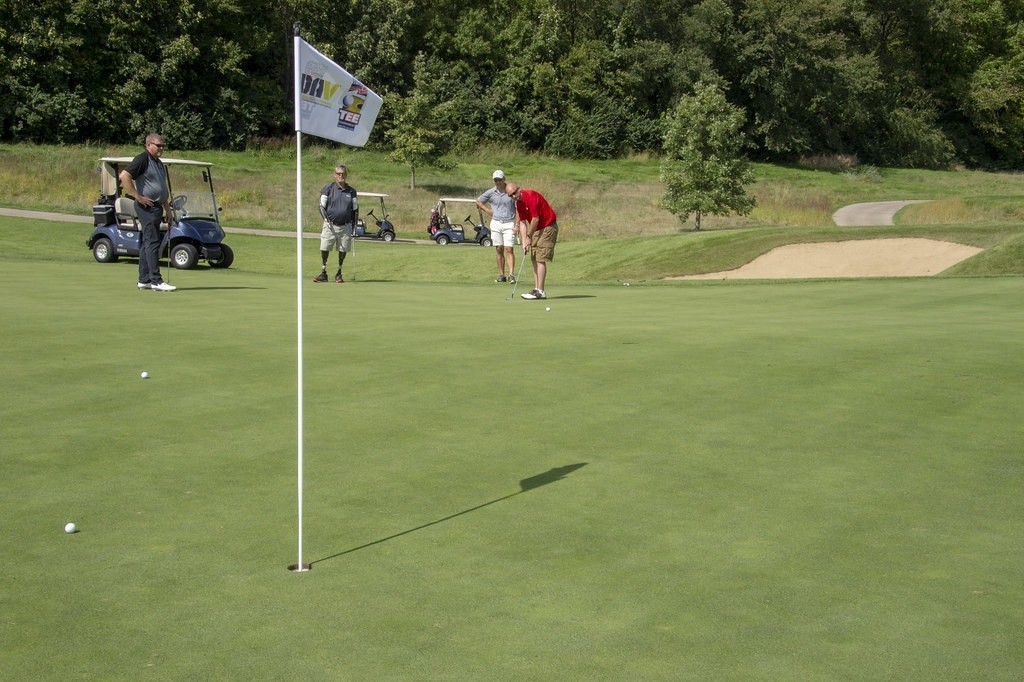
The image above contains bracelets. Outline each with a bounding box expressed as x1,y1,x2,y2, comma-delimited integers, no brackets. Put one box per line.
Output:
515,223,520,226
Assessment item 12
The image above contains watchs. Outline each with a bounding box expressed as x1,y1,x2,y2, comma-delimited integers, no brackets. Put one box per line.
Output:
527,234,533,239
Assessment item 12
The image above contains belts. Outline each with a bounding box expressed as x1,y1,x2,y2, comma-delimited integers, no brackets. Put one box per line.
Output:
330,221,350,226
139,202,161,208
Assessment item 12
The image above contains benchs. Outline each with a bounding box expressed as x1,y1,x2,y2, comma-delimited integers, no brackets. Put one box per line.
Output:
357,219,365,226
447,217,463,232
115,197,175,232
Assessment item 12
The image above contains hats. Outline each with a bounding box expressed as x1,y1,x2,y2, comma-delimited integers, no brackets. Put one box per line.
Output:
493,170,504,179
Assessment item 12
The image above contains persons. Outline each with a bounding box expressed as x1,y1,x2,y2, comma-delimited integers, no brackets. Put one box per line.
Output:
506,182,558,300
476,170,520,284
120,134,177,290
312,166,359,282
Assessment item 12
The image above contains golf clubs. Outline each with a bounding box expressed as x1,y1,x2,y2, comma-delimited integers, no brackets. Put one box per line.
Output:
350,236,355,280
166,217,172,285
506,251,528,300
516,232,528,281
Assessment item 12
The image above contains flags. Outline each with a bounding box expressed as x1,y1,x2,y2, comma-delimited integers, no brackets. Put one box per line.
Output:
294,38,383,146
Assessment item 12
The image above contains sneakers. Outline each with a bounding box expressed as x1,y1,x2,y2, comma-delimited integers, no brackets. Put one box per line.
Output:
508,275,516,284
494,276,506,282
335,273,343,282
137,281,151,289
521,288,546,299
151,282,176,291
313,273,328,282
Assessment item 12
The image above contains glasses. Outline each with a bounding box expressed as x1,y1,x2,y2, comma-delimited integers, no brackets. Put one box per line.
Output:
335,171,346,176
149,141,165,148
494,178,502,182
508,188,518,197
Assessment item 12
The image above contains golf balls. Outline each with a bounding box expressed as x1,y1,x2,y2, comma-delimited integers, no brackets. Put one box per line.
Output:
545,307,551,311
141,372,149,378
65,523,75,534
